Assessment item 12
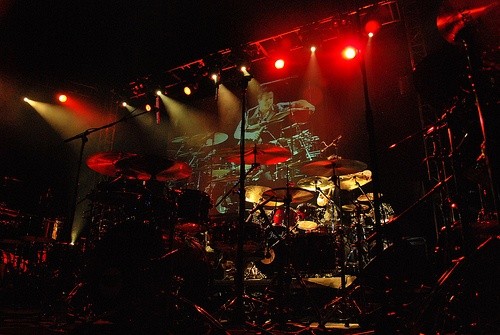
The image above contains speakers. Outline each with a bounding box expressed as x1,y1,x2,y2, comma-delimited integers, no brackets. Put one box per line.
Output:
348,234,430,321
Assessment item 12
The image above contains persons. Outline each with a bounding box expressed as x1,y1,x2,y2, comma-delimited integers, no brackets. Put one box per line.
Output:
234,86,293,140
143,159,174,195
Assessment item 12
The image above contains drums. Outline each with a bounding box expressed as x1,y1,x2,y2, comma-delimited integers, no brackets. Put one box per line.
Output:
137,180,172,234
270,137,292,162
282,233,336,275
253,226,295,277
41,215,71,243
210,223,264,263
178,189,212,221
292,129,313,155
85,221,226,331
0,202,32,236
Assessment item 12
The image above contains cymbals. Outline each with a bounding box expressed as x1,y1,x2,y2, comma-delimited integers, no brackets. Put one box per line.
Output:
224,141,293,165
262,187,314,204
86,190,136,205
244,185,284,207
114,155,192,182
215,192,239,215
86,150,144,177
298,176,333,191
357,193,383,202
341,204,368,212
337,170,372,190
189,132,228,147
301,157,367,177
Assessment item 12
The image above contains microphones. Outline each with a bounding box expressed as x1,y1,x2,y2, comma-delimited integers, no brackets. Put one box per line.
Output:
155,95,161,127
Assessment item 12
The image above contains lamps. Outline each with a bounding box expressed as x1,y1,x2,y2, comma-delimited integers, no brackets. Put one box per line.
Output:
184,81,199,95
208,64,221,82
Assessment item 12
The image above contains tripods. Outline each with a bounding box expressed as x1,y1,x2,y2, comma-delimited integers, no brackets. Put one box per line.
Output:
219,166,360,328
